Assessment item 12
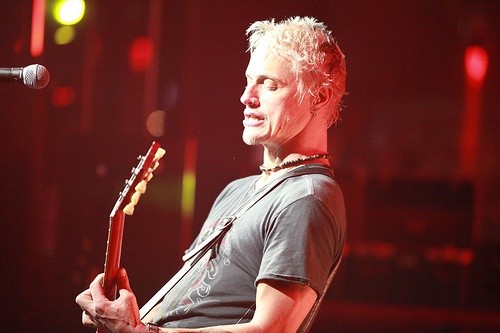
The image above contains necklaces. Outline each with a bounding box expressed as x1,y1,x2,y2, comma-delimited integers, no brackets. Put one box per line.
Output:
259,153,331,172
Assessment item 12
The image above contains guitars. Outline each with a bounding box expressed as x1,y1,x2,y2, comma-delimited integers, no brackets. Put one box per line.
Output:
96,139,167,332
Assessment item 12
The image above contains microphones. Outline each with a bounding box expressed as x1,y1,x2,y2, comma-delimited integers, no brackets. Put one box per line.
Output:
0,64,50,89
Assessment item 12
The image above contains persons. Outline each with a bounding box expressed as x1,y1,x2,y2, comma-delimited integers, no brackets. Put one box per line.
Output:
75,17,344,333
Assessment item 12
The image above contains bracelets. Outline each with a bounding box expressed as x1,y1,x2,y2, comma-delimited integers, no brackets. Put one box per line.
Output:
147,321,158,333
